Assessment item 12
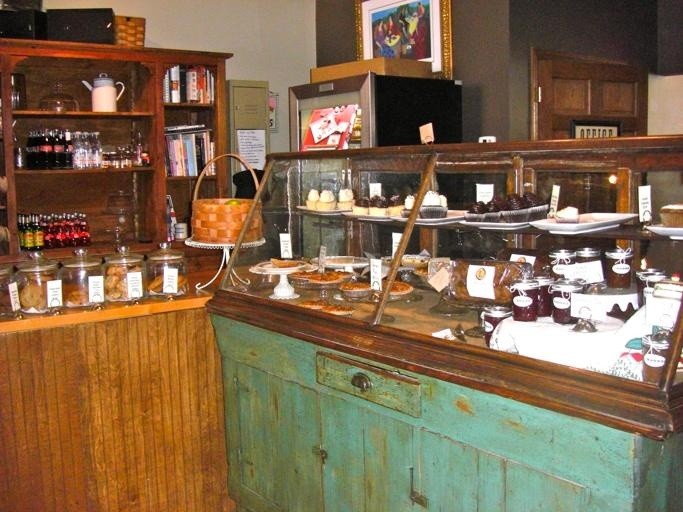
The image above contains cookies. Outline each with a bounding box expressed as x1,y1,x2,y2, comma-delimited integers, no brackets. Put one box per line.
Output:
147,273,188,293
20,275,53,312
106,267,142,299
62,283,91,304
1,288,15,314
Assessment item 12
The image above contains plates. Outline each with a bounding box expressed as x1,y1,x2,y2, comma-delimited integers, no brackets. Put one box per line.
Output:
644,220,682,244
457,221,532,231
390,210,468,226
528,212,640,235
388,283,414,301
342,288,369,300
341,211,394,222
296,204,347,219
332,293,380,302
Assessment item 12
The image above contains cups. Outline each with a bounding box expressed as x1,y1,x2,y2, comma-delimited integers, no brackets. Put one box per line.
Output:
605,250,635,290
548,277,585,325
534,275,556,317
573,245,603,295
547,247,574,302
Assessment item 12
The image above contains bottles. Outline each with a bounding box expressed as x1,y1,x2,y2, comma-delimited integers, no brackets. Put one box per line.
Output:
105,245,145,304
146,243,186,297
18,213,44,251
27,129,73,169
15,252,60,314
60,249,104,307
73,130,104,169
41,212,91,249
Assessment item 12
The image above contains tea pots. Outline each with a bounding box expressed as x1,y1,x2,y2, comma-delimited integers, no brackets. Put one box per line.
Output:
81,72,126,113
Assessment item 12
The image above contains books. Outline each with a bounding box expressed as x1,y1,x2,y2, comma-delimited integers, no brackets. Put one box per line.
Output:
163,63,216,103
163,123,217,178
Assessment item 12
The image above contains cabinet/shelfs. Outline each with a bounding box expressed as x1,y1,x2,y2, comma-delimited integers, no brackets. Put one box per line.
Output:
0,38,233,273
226,80,270,199
205,134,683,511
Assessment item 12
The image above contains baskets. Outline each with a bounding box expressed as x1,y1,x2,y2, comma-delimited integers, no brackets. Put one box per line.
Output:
191,152,262,244
114,16,145,46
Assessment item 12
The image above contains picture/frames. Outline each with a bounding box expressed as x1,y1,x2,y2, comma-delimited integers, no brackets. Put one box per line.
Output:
354,0,454,80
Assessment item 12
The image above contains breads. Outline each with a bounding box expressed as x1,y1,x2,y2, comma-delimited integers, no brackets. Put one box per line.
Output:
292,272,351,284
462,193,547,221
351,193,405,217
402,191,448,218
341,280,412,292
305,188,354,209
271,257,299,268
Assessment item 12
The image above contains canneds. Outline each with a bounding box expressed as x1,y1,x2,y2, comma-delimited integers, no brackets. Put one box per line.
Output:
635,266,671,308
175,223,187,239
605,250,632,288
535,276,553,316
551,285,572,324
642,335,671,385
547,249,577,279
103,145,151,166
577,247,605,280
